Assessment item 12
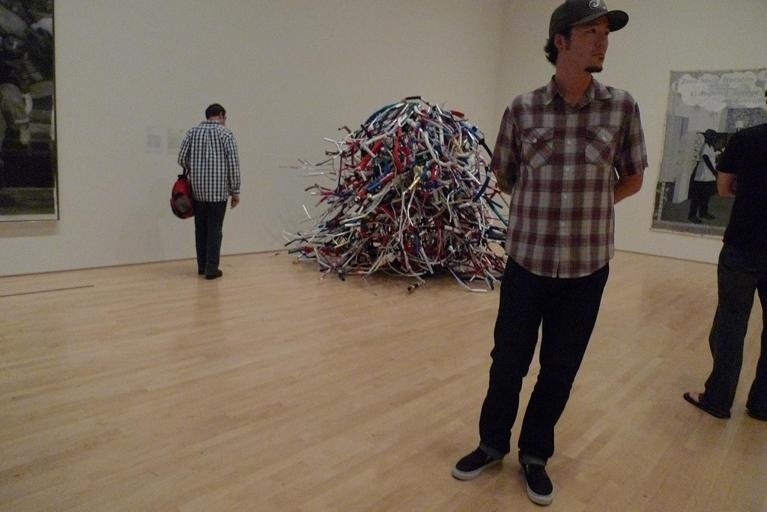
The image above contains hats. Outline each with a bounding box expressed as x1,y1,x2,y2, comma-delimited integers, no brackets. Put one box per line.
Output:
549,1,629,37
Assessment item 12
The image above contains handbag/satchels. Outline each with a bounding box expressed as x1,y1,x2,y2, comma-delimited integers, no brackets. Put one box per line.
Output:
170,174,194,219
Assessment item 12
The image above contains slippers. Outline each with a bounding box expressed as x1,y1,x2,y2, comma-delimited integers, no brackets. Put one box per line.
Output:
683,391,730,419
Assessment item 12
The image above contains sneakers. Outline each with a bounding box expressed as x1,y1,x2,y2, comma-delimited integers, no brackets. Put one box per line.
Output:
688,216,701,224
699,212,715,218
452,447,504,480
206,269,223,279
521,459,554,505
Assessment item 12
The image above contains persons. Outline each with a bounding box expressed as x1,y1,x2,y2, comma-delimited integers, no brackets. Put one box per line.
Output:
682,90,766,421
0,0,54,210
452,0,647,508
176,102,241,280
689,126,720,225
